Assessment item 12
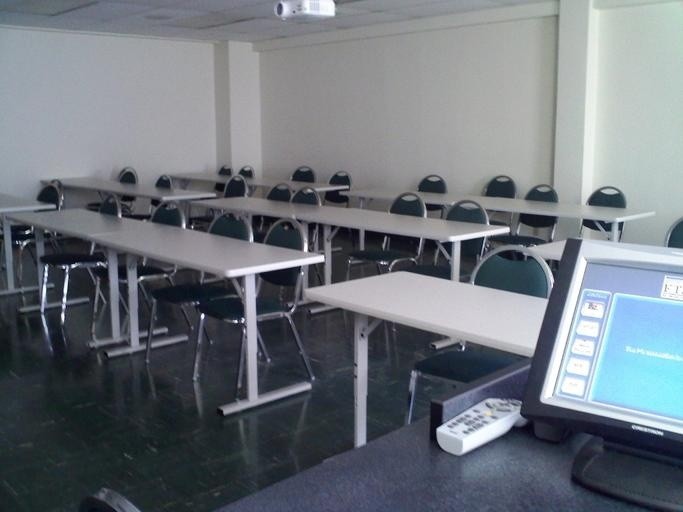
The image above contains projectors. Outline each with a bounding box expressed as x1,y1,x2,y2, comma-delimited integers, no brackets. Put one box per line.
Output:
274,0,336,20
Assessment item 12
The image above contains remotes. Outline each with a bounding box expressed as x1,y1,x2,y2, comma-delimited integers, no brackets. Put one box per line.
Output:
436,397,522,457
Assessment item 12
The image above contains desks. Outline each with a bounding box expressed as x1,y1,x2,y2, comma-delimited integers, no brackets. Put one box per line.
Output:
302,270,548,446
90,225,326,418
0,206,174,349
524,238,683,264
188,196,343,310
40,176,219,216
170,173,351,205
217,358,683,512
295,202,511,351
341,190,657,241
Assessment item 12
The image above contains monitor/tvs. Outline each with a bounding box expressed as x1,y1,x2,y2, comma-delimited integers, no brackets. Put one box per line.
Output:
519,236,683,512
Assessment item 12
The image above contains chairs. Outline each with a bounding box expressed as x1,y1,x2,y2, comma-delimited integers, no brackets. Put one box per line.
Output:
664,217,683,249
237,164,256,195
415,174,448,217
39,192,123,321
322,170,352,208
124,172,172,220
91,199,185,336
212,163,234,196
0,178,64,289
477,174,516,229
404,245,554,427
515,183,560,239
191,217,316,388
86,165,138,214
137,210,254,366
576,185,627,243
186,174,248,229
345,192,427,280
291,166,315,183
254,182,292,243
432,200,489,283
289,186,324,286
